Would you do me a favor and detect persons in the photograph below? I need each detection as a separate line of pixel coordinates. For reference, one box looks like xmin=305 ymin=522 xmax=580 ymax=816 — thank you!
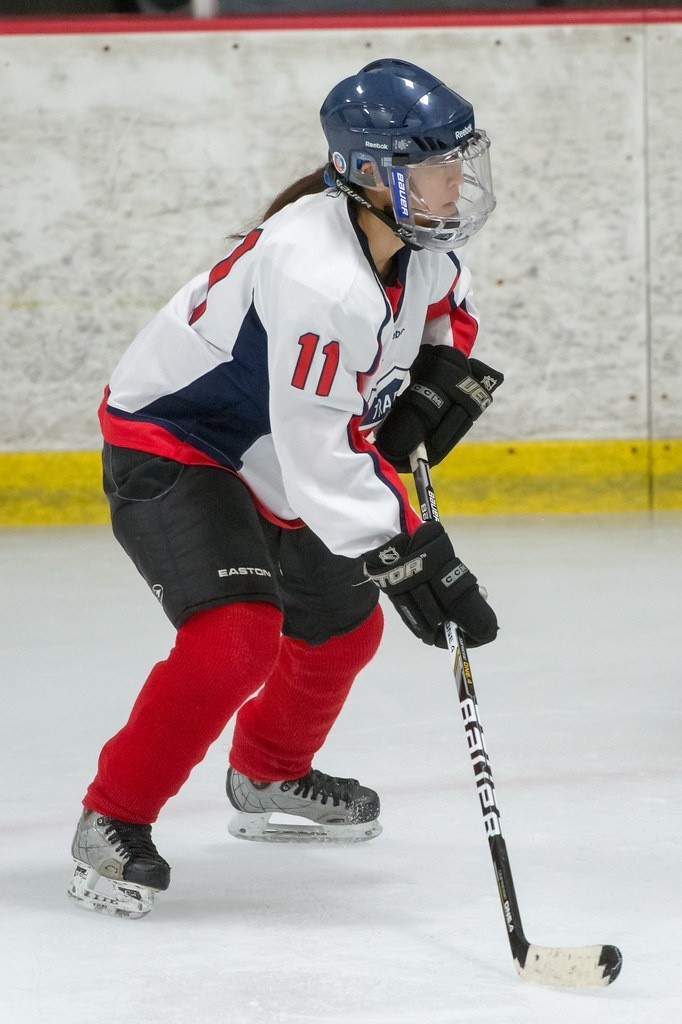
xmin=70 ymin=58 xmax=503 ymax=890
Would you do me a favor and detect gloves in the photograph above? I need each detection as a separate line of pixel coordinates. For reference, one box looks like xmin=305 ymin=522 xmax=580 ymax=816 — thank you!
xmin=373 ymin=344 xmax=505 ymax=475
xmin=364 ymin=522 xmax=499 ymax=649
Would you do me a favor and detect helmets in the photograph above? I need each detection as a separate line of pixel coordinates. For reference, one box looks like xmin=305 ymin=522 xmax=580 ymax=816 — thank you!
xmin=320 ymin=59 xmax=498 ymax=254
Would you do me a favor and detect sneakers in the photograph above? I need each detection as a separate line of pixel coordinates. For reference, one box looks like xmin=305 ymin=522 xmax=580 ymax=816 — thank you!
xmin=226 ymin=765 xmax=383 ymax=849
xmin=67 ymin=804 xmax=172 ymax=920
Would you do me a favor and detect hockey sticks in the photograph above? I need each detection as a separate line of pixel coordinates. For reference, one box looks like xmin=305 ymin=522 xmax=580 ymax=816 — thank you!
xmin=409 ymin=446 xmax=625 ymax=994
